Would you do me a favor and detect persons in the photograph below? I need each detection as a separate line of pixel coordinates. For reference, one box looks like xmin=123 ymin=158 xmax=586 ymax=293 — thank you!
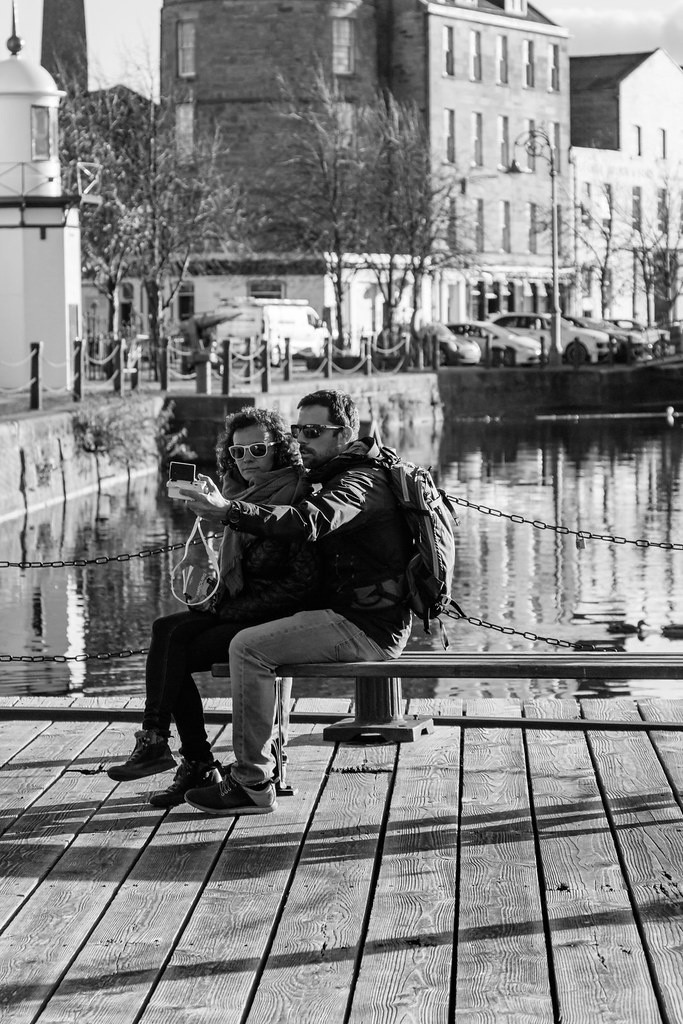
xmin=109 ymin=408 xmax=320 ymax=808
xmin=180 ymin=389 xmax=413 ymax=815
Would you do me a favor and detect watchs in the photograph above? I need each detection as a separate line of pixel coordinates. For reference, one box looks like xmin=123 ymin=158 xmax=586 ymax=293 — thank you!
xmin=226 ymin=501 xmax=240 ymax=526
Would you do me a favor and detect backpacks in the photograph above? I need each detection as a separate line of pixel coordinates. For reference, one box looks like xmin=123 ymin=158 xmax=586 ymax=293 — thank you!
xmin=378 ymin=444 xmax=468 ymax=653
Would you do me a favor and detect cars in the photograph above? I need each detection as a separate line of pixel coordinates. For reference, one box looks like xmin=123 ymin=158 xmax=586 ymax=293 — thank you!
xmin=564 ymin=316 xmax=653 ymax=361
xmin=447 ymin=322 xmax=543 ymax=366
xmin=491 ymin=313 xmax=618 ymax=364
xmin=609 ymin=319 xmax=672 ymax=359
xmin=364 ymin=323 xmax=481 ymax=366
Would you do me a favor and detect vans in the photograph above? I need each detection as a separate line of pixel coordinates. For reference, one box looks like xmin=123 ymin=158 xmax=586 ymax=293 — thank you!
xmin=219 ymin=298 xmax=333 ymax=373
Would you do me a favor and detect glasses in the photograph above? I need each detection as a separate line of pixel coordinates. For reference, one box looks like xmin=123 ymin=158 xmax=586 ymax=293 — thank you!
xmin=228 ymin=441 xmax=278 ymax=460
xmin=291 ymin=424 xmax=343 ymax=439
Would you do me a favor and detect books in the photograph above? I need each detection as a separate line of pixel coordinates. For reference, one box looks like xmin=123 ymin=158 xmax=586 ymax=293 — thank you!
xmin=182 ymin=565 xmax=224 ymax=614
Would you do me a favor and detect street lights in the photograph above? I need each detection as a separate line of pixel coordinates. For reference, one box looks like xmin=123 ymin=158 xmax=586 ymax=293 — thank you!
xmin=517 ymin=131 xmax=564 ymax=354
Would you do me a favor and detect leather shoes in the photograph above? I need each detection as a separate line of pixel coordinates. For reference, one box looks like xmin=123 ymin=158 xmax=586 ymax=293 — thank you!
xmin=108 ymin=732 xmax=177 ymax=781
xmin=150 ymin=758 xmax=223 ymax=808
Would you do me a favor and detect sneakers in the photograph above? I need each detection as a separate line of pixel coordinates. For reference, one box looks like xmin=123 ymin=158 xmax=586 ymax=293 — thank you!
xmin=184 ymin=762 xmax=273 ymax=816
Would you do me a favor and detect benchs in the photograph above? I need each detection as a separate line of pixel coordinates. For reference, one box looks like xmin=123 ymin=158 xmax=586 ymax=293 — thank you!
xmin=212 ymin=650 xmax=683 ymax=796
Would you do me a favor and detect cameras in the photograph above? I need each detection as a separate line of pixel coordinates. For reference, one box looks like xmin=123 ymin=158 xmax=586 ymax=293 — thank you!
xmin=165 ymin=461 xmax=208 ymax=502
